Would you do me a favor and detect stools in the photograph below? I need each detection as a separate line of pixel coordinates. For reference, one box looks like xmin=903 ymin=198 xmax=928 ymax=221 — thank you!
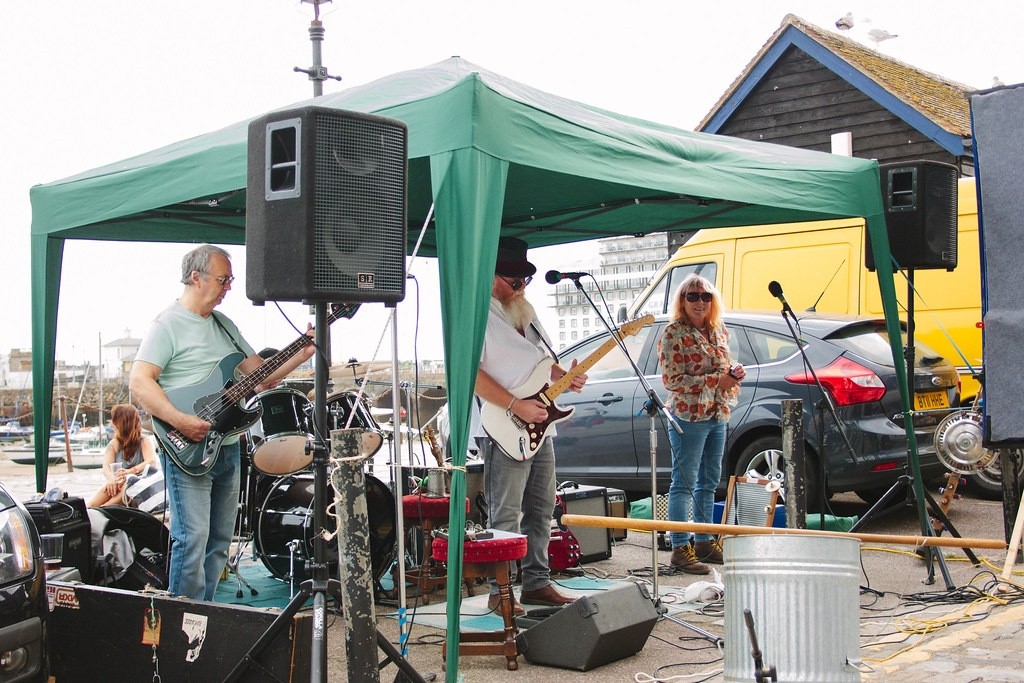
xmin=391 ymin=492 xmax=475 ymax=601
xmin=431 ymin=530 xmax=528 ymax=669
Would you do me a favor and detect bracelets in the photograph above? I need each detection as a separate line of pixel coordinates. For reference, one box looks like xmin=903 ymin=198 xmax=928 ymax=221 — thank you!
xmin=560 ymin=372 xmax=566 ymax=378
xmin=506 ymin=396 xmax=516 ymax=411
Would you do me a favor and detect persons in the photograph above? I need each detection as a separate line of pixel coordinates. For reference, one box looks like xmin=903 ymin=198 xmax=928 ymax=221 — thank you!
xmin=231 ymin=347 xmax=315 ymax=542
xmin=656 ymin=273 xmax=747 ymax=574
xmin=474 ymin=237 xmax=588 ymax=616
xmin=128 ymin=245 xmax=315 ymax=602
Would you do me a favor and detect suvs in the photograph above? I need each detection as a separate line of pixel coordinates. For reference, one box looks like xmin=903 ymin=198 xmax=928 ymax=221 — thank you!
xmin=435 ymin=310 xmax=965 ymax=514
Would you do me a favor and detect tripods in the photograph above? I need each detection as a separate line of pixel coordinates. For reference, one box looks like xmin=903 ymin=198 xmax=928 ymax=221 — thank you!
xmin=222 ymin=302 xmax=426 ymax=683
xmin=846 ymin=269 xmax=983 ymax=586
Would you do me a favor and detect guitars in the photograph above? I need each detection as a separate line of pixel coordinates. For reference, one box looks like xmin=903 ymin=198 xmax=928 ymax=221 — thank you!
xmin=150 ymin=303 xmax=363 ymax=478
xmin=480 ymin=310 xmax=656 ymax=463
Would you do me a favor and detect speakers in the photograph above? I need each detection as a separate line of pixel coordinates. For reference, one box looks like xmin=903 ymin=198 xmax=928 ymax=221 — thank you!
xmin=245 ymin=105 xmax=407 ymax=308
xmin=556 ymin=484 xmax=612 ymax=563
xmin=515 ymin=580 xmax=658 ymax=671
xmin=865 ymin=160 xmax=960 ymax=273
xmin=606 ymin=488 xmax=628 ymax=541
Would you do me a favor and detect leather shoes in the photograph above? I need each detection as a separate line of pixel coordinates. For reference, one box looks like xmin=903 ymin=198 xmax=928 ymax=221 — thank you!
xmin=520 ymin=584 xmax=578 ymax=607
xmin=487 ymin=590 xmax=525 ymax=616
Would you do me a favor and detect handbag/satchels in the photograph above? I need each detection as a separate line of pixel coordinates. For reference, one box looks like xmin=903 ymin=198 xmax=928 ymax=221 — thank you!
xmin=121 ymin=464 xmax=171 ymax=515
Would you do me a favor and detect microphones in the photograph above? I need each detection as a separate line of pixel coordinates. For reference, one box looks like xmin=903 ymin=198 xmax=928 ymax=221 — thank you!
xmin=545 ymin=270 xmax=589 ymax=284
xmin=768 ymin=281 xmax=798 ymax=322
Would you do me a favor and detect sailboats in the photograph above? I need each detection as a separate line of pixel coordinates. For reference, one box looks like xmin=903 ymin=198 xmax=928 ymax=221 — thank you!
xmin=1 ymin=332 xmax=159 ymax=470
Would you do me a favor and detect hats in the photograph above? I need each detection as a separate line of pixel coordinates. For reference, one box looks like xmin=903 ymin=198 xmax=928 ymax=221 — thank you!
xmin=495 ymin=236 xmax=537 ymax=278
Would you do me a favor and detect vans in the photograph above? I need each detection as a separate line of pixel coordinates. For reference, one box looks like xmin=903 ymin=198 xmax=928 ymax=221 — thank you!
xmin=618 ymin=176 xmax=1023 ymax=499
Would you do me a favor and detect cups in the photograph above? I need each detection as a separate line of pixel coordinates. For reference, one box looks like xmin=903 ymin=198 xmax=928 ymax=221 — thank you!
xmin=40 ymin=534 xmax=65 ymax=571
xmin=109 ymin=462 xmax=123 ymax=484
xmin=426 ymin=469 xmax=446 ymax=498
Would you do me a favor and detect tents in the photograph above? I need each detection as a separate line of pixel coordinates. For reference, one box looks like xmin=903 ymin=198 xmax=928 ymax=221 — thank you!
xmin=29 ymin=56 xmax=936 ymax=683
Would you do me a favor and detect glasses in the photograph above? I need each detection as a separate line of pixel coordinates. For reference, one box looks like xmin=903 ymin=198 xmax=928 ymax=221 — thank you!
xmin=199 ymin=270 xmax=235 ymax=286
xmin=496 ymin=272 xmax=533 ymax=290
xmin=683 ymin=291 xmax=712 ymax=303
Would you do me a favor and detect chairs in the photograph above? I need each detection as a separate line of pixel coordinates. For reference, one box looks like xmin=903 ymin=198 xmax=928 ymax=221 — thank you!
xmin=777 ymin=345 xmax=798 ymax=359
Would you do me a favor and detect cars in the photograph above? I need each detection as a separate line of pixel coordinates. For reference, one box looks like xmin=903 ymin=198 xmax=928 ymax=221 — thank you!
xmin=0 ymin=483 xmax=51 ymax=683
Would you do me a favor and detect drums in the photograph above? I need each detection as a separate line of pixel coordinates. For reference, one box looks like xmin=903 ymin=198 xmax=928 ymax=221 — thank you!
xmin=243 ymin=387 xmax=316 ymax=477
xmin=326 ymin=390 xmax=384 ymax=463
xmin=252 ymin=465 xmax=397 ymax=590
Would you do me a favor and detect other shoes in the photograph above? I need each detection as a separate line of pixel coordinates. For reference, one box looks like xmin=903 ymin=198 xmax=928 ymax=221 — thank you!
xmin=669 ymin=543 xmax=711 ymax=574
xmin=693 ymin=539 xmax=724 ymax=564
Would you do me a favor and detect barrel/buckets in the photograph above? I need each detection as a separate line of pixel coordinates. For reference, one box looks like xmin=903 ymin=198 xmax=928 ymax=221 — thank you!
xmin=722 ymin=533 xmax=875 ymax=683
